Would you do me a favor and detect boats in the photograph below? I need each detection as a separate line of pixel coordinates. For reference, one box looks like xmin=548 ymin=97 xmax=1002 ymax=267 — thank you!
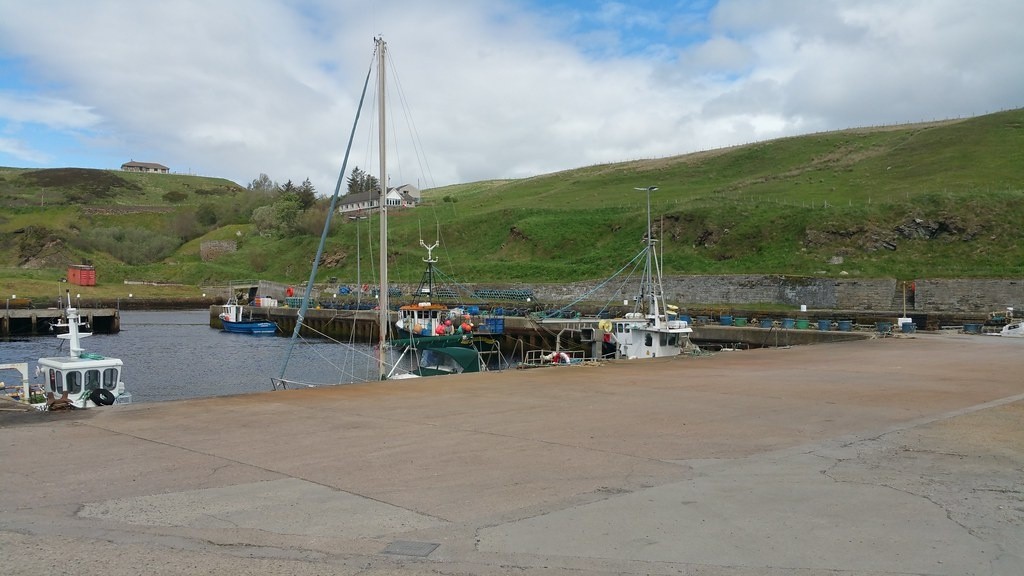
xmin=219 ymin=280 xmax=278 ymax=333
xmin=0 ymin=281 xmax=133 ymax=412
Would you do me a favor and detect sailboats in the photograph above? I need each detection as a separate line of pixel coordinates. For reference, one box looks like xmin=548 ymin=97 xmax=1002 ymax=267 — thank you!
xmin=269 ymin=34 xmax=749 ymax=390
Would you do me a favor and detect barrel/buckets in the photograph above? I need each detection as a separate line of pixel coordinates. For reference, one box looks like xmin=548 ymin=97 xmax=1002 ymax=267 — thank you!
xmin=818 ymin=320 xmax=832 ymax=331
xmin=679 ymin=316 xmax=690 ymax=324
xmin=838 ymin=320 xmax=852 ymax=331
xmin=796 ymin=320 xmax=809 ymax=329
xmin=720 ymin=317 xmax=732 ymax=326
xmin=782 ymin=319 xmax=794 ymax=328
xmin=964 ymin=323 xmax=980 ymax=332
xmin=759 ymin=319 xmax=772 ymax=328
xmin=735 ymin=318 xmax=747 ymax=327
xmin=902 ymin=322 xmax=916 ymax=332
xmin=878 ymin=321 xmax=891 ymax=332
